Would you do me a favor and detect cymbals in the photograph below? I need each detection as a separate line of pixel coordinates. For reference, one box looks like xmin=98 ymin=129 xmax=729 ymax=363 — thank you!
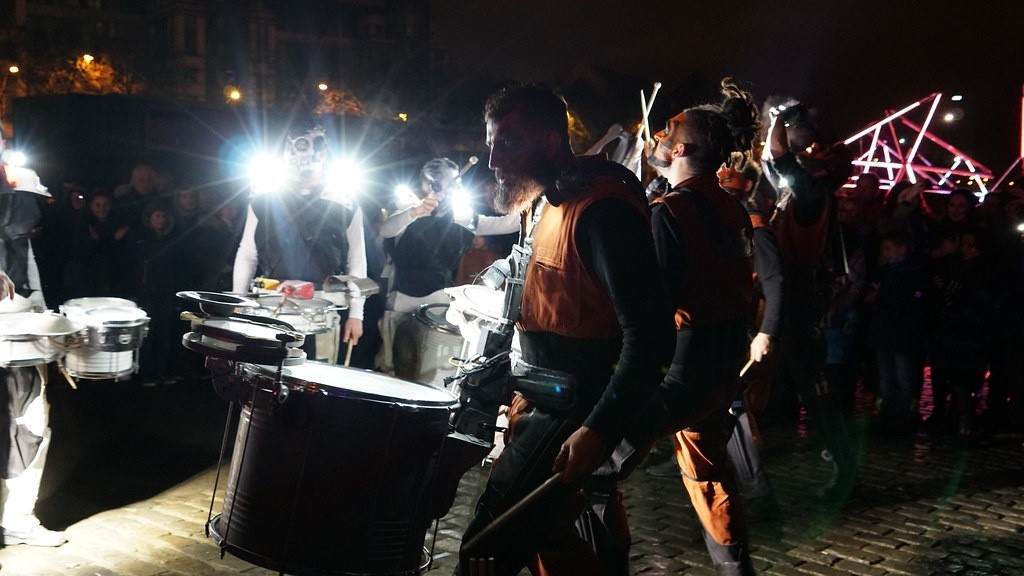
xmin=25 ymin=311 xmax=83 ymax=336
xmin=176 ymin=291 xmax=261 ymax=316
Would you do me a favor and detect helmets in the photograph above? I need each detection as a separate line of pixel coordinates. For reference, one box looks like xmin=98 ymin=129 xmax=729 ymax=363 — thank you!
xmin=1 ymin=166 xmax=51 ymax=197
xmin=422 ymin=158 xmax=463 ymax=194
xmin=285 ymin=124 xmax=332 ymax=180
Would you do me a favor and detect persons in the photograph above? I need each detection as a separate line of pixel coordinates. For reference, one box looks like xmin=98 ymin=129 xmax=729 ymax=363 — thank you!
xmin=452 ymin=84 xmax=678 ymax=576
xmin=0 ymin=77 xmax=1024 ymax=576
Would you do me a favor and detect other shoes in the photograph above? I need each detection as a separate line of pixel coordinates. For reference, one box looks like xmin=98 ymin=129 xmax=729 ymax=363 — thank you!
xmin=824 ymin=475 xmax=840 ymax=492
xmin=646 ymin=459 xmax=682 ymax=477
xmin=750 ymin=494 xmax=777 ymax=518
xmin=0 ymin=526 xmax=67 ymax=547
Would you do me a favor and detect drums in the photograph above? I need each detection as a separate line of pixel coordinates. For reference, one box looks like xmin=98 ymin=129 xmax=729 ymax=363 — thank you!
xmin=409 ymin=303 xmax=518 ymax=449
xmin=57 ymin=296 xmax=144 ymax=381
xmin=234 ymin=295 xmax=342 ymax=365
xmin=203 ymin=358 xmax=464 ymax=576
xmin=0 ymin=311 xmax=66 ymax=369
xmin=181 ymin=314 xmax=308 ymax=366
xmin=80 ymin=304 xmax=151 ymax=353
